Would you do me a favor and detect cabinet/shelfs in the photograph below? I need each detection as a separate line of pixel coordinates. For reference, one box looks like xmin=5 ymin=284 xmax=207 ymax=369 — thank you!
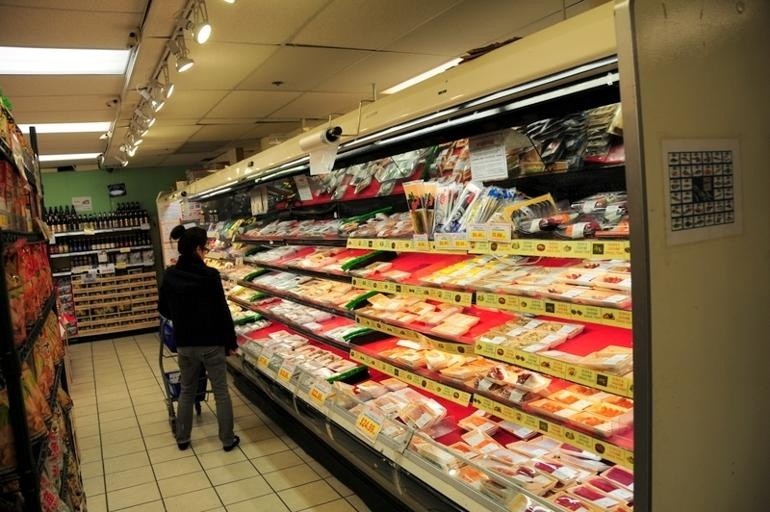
xmin=46 ymin=201 xmax=161 ymax=341
xmin=0 ymin=133 xmax=86 ymax=512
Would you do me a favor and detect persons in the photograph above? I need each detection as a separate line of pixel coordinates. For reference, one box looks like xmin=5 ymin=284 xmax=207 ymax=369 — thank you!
xmin=157 ymin=225 xmax=239 ymax=452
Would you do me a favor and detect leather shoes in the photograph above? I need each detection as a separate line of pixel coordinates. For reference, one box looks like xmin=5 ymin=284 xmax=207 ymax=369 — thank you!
xmin=222 ymin=434 xmax=240 ymax=452
xmin=177 ymin=440 xmax=192 ymax=450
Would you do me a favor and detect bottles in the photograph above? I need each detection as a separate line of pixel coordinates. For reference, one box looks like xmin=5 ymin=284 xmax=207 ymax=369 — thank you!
xmin=41 ymin=200 xmax=151 ymax=272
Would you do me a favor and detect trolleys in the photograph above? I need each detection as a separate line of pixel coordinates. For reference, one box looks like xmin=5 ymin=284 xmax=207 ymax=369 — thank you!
xmin=159 ymin=315 xmax=207 ymax=418
